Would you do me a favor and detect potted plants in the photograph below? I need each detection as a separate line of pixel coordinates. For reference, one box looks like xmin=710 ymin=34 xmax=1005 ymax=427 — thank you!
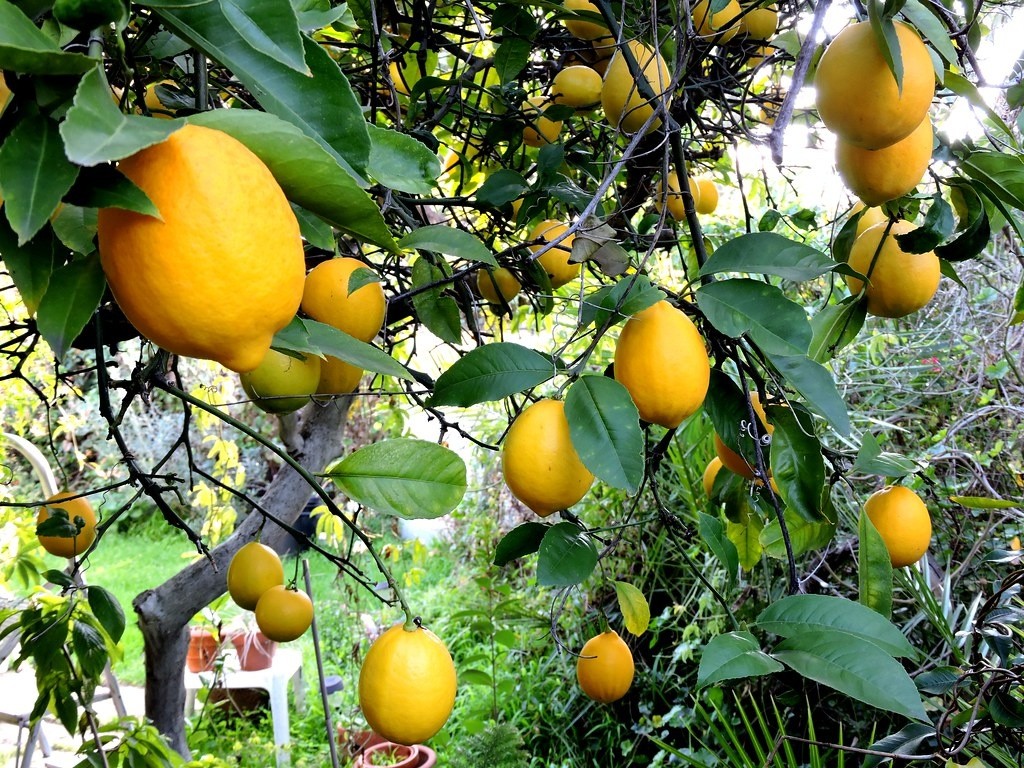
xmin=358 ymin=741 xmax=420 ymax=768
xmin=218 ymin=596 xmax=277 ymax=671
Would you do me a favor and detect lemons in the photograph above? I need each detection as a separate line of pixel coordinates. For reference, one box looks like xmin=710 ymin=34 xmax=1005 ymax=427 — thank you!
xmin=37 ymin=0 xmax=939 ymax=746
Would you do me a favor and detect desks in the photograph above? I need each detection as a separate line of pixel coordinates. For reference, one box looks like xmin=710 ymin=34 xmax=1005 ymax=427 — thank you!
xmin=184 ymin=648 xmax=305 ymax=768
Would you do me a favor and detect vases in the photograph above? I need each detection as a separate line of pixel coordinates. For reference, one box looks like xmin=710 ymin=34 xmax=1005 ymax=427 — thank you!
xmin=353 ymin=744 xmax=438 ymax=768
